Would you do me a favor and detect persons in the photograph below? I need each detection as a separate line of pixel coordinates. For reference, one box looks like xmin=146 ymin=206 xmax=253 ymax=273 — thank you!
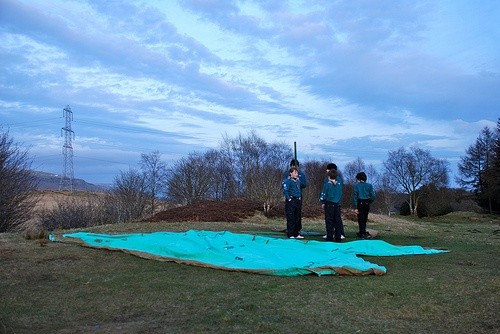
xmin=281 ymin=167 xmax=306 ymax=239
xmin=280 ymin=158 xmax=308 ymax=233
xmin=318 ymin=169 xmax=344 ymax=243
xmin=321 ymin=162 xmax=347 ymax=240
xmin=353 ymin=172 xmax=375 ymax=239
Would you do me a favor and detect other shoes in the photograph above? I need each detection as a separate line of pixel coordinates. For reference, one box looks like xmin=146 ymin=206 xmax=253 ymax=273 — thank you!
xmin=296 ymin=235 xmax=305 ymax=238
xmin=360 ymin=232 xmax=372 ymax=240
xmin=322 ymin=234 xmax=335 ymax=239
xmin=290 ymin=236 xmax=295 ymax=239
xmin=340 ymin=235 xmax=346 ymax=239
